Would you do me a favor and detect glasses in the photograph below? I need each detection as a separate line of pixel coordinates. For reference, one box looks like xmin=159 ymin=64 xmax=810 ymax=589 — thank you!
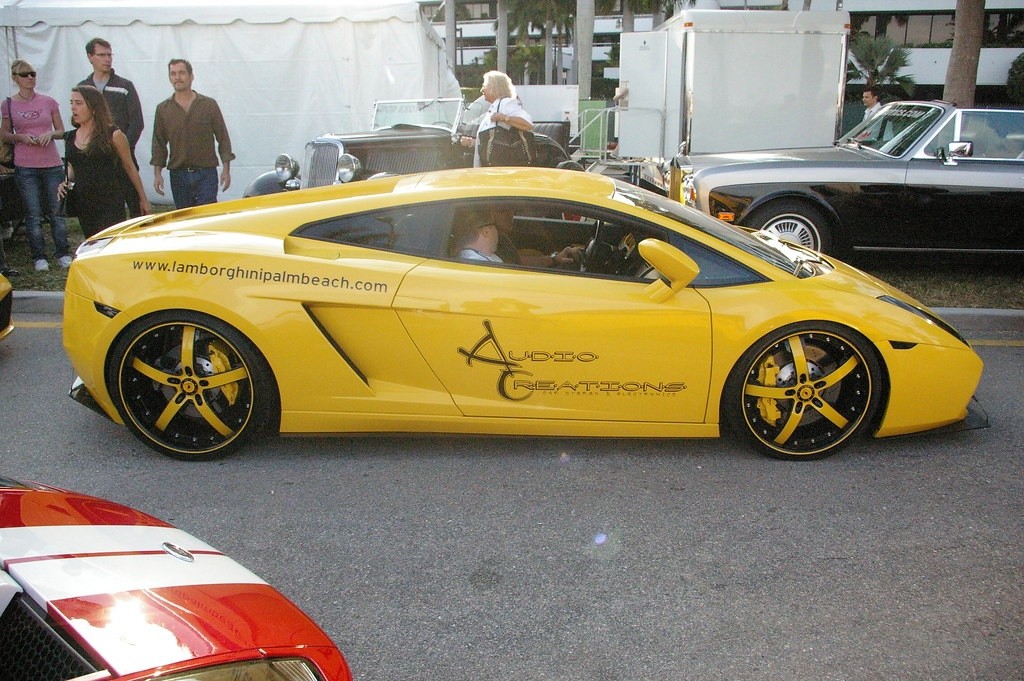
xmin=95 ymin=53 xmax=115 ymax=60
xmin=471 ymin=221 xmax=500 ymax=231
xmin=17 ymin=71 xmax=36 ymax=78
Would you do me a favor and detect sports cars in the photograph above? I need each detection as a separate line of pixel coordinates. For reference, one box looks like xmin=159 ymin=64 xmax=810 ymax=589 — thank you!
xmin=60 ymin=166 xmax=986 ymax=462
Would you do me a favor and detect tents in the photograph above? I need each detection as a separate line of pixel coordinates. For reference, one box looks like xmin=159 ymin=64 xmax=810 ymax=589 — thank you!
xmin=0 ymin=3 xmax=463 ymax=208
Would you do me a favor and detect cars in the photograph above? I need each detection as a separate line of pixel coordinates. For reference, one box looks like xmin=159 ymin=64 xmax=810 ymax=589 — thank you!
xmin=671 ymin=99 xmax=1023 ymax=264
xmin=299 ymin=120 xmax=571 ymax=190
xmin=0 ymin=470 xmax=351 ymax=680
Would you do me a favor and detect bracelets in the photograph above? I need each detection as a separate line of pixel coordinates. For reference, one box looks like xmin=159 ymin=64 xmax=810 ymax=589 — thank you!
xmin=468 ymin=137 xmax=474 ymax=148
xmin=11 ymin=134 xmax=17 ymax=144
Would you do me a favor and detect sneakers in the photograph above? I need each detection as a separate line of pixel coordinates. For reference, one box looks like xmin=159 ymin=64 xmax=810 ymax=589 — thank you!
xmin=58 ymin=255 xmax=73 ymax=269
xmin=33 ymin=259 xmax=50 ymax=272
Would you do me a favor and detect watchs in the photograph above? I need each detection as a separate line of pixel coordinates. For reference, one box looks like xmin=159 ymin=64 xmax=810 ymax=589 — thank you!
xmin=550 ymin=251 xmax=561 ymax=264
xmin=505 ymin=115 xmax=510 ymax=124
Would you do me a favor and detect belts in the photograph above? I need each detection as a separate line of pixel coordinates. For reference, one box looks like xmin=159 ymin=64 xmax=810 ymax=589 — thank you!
xmin=170 ymin=165 xmax=216 ymax=173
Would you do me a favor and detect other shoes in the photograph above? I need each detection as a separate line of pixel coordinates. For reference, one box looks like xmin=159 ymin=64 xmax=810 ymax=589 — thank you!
xmin=1 ymin=268 xmax=20 ymax=277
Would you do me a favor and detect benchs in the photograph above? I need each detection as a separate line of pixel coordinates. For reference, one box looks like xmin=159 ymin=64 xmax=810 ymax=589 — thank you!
xmin=457 ymin=120 xmax=571 ymax=152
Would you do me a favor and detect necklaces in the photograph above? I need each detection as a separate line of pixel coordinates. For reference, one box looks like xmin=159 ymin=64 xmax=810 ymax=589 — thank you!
xmin=18 ymin=91 xmax=37 ymax=102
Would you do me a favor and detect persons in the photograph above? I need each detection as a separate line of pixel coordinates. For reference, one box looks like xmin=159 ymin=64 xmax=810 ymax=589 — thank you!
xmin=863 ymin=87 xmax=893 ymax=143
xmin=461 ymin=70 xmax=532 ymax=168
xmin=452 ymin=201 xmax=582 ymax=266
xmin=0 ymin=38 xmax=236 ymax=271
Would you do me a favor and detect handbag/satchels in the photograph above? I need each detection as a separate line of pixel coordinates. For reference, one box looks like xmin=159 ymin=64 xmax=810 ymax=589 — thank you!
xmin=478 ymin=96 xmax=540 ymax=167
xmin=0 ymin=96 xmax=14 ymax=169
xmin=53 ymin=131 xmax=78 ymax=218
